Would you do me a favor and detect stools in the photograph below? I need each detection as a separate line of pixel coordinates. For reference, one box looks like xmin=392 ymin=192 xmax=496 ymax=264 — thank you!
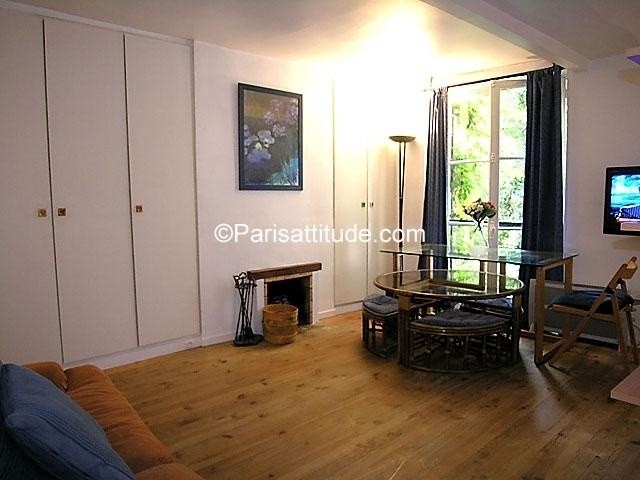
xmin=360 ymin=291 xmax=524 ymax=375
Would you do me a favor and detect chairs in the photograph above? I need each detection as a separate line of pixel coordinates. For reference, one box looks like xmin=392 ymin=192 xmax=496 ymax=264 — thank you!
xmin=542 ymin=256 xmax=640 ymax=375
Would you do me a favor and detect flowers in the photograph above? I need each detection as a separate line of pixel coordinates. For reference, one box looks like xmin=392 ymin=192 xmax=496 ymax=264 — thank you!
xmin=462 ymin=197 xmax=498 ymax=246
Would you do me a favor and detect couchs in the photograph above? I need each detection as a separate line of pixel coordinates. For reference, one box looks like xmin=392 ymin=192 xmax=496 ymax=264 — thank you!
xmin=0 ymin=361 xmax=205 ymax=480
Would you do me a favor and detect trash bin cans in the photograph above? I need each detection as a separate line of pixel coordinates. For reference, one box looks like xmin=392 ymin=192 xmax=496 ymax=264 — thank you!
xmin=262 ymin=304 xmax=298 ymax=344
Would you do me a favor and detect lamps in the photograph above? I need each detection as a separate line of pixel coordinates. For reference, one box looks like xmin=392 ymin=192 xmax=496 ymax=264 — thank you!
xmin=389 ymin=135 xmax=416 ymax=286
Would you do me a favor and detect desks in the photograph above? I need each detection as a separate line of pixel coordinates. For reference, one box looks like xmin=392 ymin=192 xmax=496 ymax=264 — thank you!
xmin=373 ymin=268 xmax=526 ymax=372
xmin=380 ymin=242 xmax=580 ymax=366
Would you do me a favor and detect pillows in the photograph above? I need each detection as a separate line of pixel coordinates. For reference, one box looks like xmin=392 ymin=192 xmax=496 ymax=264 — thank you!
xmin=2 ymin=362 xmax=135 ymax=480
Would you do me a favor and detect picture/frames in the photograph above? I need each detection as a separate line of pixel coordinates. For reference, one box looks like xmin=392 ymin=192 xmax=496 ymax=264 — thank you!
xmin=237 ymin=82 xmax=303 ymax=191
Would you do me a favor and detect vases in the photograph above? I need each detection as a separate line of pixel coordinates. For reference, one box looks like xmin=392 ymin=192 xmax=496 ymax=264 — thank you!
xmin=466 ymin=220 xmax=489 ymax=258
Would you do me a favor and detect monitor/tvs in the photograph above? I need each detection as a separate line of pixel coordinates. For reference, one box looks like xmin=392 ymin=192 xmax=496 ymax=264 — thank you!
xmin=602 ymin=165 xmax=640 ymax=237
